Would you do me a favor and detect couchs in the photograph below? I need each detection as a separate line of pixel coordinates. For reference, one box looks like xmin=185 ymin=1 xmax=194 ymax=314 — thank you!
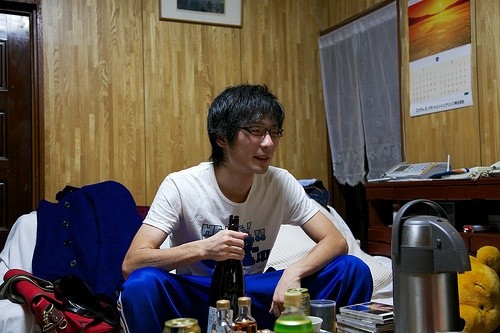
xmin=0 ymin=181 xmax=394 ymax=333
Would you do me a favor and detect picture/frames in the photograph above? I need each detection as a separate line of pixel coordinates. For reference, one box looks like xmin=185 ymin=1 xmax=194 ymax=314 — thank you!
xmin=159 ymin=0 xmax=243 ymax=28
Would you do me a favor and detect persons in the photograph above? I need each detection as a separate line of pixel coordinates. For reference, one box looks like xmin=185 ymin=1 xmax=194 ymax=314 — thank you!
xmin=116 ymin=83 xmax=374 ymax=332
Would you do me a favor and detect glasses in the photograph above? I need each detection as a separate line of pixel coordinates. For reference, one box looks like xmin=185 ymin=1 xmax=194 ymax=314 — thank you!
xmin=236 ymin=124 xmax=284 ymax=138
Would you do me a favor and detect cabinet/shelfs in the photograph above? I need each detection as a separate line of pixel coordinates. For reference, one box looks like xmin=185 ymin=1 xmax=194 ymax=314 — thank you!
xmin=365 ymin=177 xmax=500 ymax=258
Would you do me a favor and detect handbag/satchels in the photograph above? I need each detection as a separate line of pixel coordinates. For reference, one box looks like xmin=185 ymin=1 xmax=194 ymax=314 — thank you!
xmin=0 ymin=269 xmax=121 ymax=333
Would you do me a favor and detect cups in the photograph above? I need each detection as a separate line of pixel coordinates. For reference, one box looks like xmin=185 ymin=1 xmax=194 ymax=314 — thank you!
xmin=307 ymin=316 xmax=323 ymax=333
xmin=309 ymin=300 xmax=337 ymax=333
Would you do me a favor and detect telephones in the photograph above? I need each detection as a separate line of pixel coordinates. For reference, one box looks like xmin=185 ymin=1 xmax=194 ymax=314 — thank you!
xmin=470 ymin=160 xmax=500 ymax=180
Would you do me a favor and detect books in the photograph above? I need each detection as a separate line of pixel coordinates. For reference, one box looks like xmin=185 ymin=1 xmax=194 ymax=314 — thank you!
xmin=335 ymin=301 xmax=394 ymax=333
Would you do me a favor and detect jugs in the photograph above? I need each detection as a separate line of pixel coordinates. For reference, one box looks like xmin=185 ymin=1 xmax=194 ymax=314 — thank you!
xmin=389 ymin=198 xmax=473 ymax=333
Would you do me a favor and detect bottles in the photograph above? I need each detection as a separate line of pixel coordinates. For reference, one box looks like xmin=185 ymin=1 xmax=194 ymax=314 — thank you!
xmin=211 ymin=300 xmax=237 ymax=333
xmin=272 ymin=290 xmax=315 ymax=333
xmin=207 ymin=214 xmax=246 ymax=333
xmin=232 ymin=297 xmax=257 ymax=333
